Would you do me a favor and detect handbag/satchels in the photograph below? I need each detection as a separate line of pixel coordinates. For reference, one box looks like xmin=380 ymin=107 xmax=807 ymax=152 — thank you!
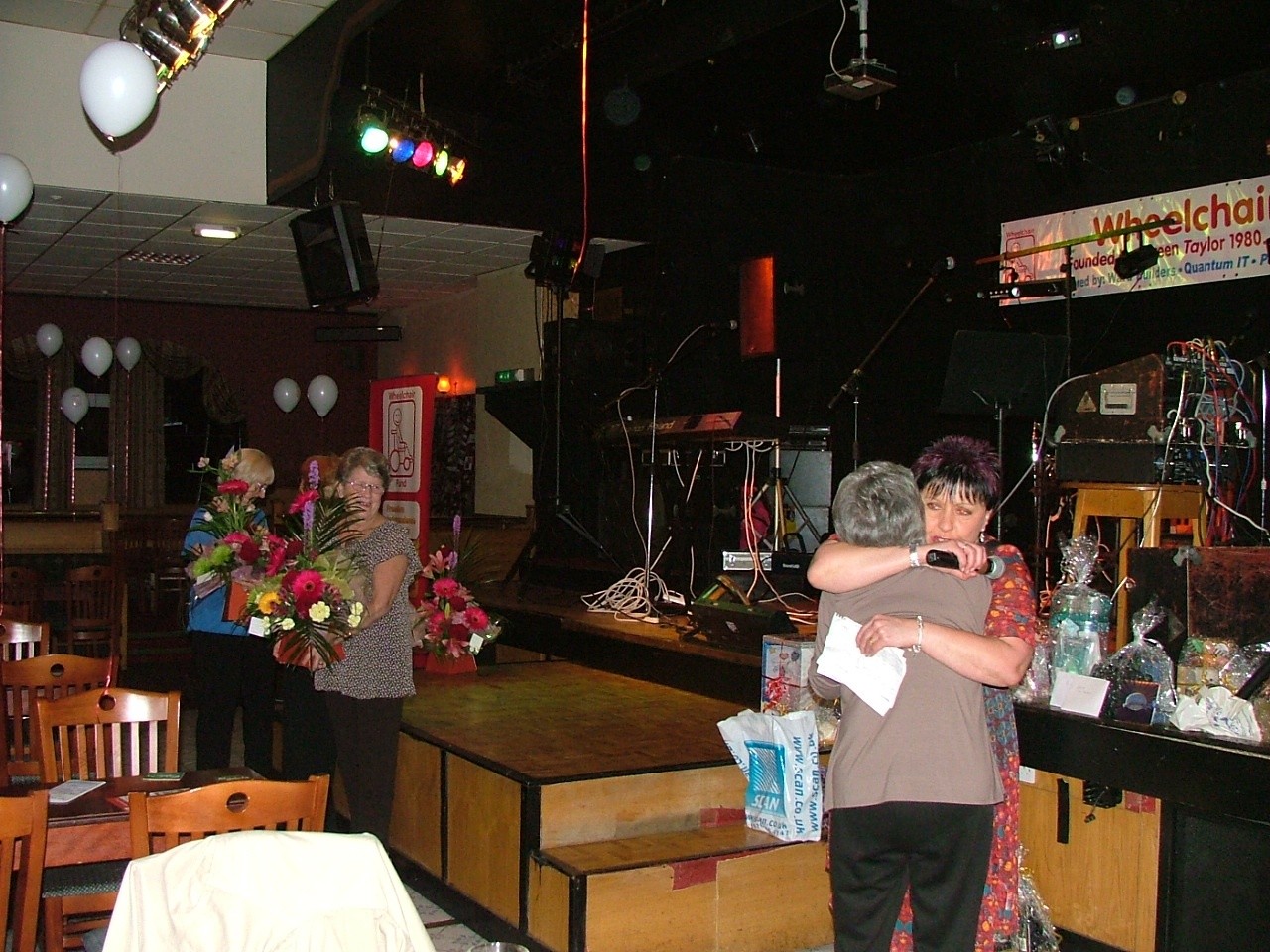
xmin=718 ymin=710 xmax=823 ymax=842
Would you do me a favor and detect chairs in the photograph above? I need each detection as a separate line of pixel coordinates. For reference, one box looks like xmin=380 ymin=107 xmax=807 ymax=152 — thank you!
xmin=0 ymin=526 xmax=330 ymax=952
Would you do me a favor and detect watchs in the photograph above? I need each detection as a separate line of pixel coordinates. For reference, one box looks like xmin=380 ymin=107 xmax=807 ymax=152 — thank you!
xmin=909 ymin=541 xmax=921 ymax=567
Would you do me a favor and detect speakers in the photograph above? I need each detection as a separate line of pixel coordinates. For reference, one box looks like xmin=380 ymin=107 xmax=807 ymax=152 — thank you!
xmin=291 ymin=200 xmax=381 ymax=310
xmin=765 ymin=445 xmax=833 ymax=555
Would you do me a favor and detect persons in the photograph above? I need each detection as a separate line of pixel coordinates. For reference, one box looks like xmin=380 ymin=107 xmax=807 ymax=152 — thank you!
xmin=280 ymin=448 xmax=425 ymax=850
xmin=182 ymin=449 xmax=276 ymax=771
xmin=806 ymin=437 xmax=1060 ymax=951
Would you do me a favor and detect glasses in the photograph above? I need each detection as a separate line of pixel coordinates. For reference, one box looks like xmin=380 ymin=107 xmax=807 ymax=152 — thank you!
xmin=344 ymin=480 xmax=386 ymax=496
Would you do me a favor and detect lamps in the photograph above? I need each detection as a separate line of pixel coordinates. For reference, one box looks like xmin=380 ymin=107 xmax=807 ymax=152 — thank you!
xmin=502 ymin=228 xmax=606 ymax=602
xmin=436 ymin=375 xmax=451 ymax=398
xmin=357 ymin=92 xmax=467 ymax=187
xmin=1114 ymin=232 xmax=1159 ymax=279
xmin=120 ymin=0 xmax=235 ymax=95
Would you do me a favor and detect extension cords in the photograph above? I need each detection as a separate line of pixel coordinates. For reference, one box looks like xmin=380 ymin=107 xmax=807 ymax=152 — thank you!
xmin=625 ymin=612 xmax=659 ymax=623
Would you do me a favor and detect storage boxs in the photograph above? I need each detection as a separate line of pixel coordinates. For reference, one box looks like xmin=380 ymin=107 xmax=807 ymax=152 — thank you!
xmin=761 ymin=633 xmax=841 ymax=745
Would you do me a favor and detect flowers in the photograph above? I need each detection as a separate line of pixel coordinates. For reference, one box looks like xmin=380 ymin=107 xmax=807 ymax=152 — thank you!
xmin=176 ymin=445 xmax=371 ymax=677
xmin=411 ymin=525 xmax=511 ymax=667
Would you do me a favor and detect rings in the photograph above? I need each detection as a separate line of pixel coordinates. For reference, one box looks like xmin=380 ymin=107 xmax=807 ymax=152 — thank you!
xmin=962 ymin=544 xmax=968 ymax=551
xmin=870 ymin=636 xmax=874 ymax=644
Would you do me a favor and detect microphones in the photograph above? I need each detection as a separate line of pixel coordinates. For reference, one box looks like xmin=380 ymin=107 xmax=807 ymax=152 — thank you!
xmin=927 ymin=550 xmax=1005 ymax=579
xmin=706 ymin=321 xmax=737 ymax=330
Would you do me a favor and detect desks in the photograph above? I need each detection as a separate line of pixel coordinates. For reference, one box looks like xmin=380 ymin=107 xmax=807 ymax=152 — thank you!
xmin=3 ymin=580 xmax=108 ymax=601
xmin=1057 ymin=480 xmax=1209 ymax=651
xmin=6 ymin=685 xmax=85 ymax=720
xmin=0 ymin=766 xmax=268 ymax=871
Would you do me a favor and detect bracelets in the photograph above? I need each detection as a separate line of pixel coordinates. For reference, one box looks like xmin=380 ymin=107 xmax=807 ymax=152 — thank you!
xmin=907 ymin=615 xmax=923 ymax=655
